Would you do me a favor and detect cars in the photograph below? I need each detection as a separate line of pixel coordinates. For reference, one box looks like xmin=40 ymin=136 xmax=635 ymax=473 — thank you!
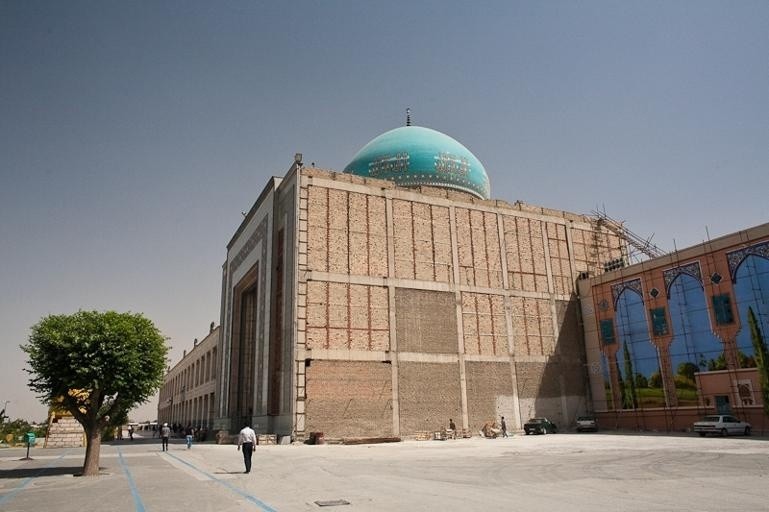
xmin=693 ymin=414 xmax=752 ymax=436
xmin=523 ymin=417 xmax=557 ymax=435
xmin=575 ymin=414 xmax=599 ymax=432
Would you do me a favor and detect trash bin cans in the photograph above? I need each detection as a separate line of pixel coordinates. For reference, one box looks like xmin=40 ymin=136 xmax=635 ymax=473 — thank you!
xmin=24 ymin=432 xmax=35 ymax=447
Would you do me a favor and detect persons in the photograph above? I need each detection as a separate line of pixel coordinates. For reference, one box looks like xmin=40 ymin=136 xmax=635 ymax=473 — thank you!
xmin=236 ymin=420 xmax=257 ymax=476
xmin=449 ymin=418 xmax=457 ymax=439
xmin=500 ymin=416 xmax=508 ymax=438
xmin=127 ymin=421 xmax=202 ymax=452
